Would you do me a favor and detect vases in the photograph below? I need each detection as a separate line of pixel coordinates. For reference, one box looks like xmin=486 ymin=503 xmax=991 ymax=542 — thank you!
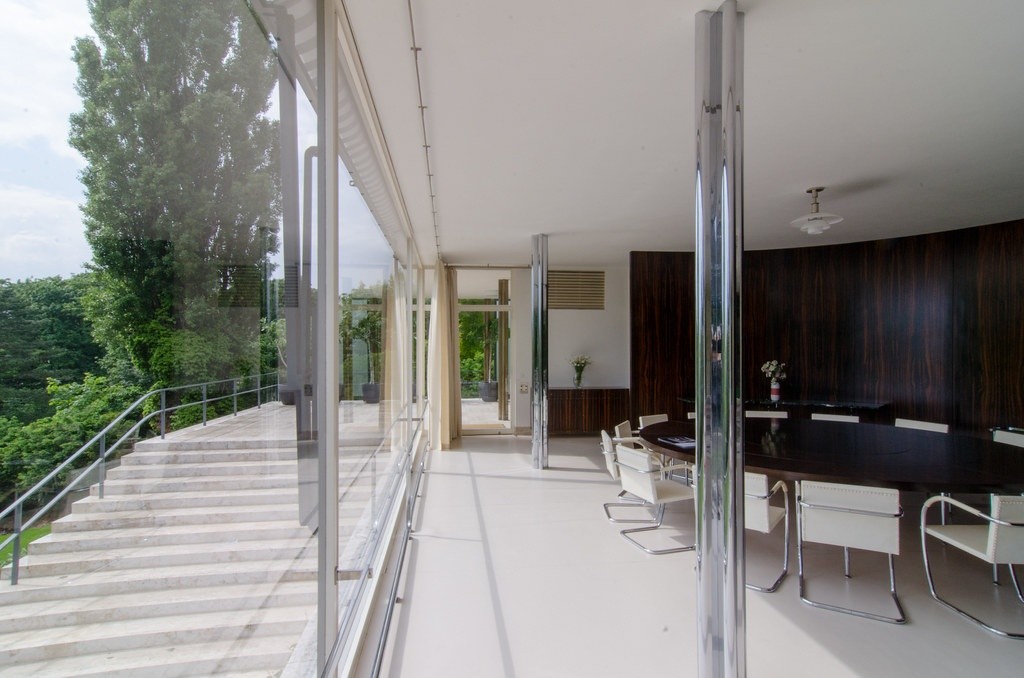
xmin=571 ymin=366 xmax=585 ymax=387
xmin=770 ymin=382 xmax=781 ymax=401
xmin=278 ymin=385 xmax=300 ymax=406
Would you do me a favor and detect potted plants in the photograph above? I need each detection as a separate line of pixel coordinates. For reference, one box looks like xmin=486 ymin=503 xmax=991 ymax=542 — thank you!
xmin=350 ymin=313 xmax=382 ymax=403
xmin=470 ymin=320 xmax=498 ymax=402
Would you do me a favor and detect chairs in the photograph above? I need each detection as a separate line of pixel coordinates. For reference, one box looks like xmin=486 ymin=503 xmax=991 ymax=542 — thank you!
xmin=602 ymin=411 xmax=1024 ymax=640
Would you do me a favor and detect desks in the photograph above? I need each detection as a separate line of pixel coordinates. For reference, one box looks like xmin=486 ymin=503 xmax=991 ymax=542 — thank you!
xmin=639 ymin=418 xmax=1024 ymax=496
xmin=531 ymin=387 xmax=631 ymax=437
xmin=676 ymin=392 xmax=893 ymax=425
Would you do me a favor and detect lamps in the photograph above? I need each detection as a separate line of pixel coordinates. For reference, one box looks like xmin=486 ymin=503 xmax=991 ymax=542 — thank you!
xmin=790 ymin=187 xmax=845 ymax=235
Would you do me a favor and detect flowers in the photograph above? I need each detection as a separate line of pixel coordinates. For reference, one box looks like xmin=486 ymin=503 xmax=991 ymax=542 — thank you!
xmin=567 ymin=353 xmax=593 ymax=384
xmin=760 ymin=361 xmax=787 ymax=381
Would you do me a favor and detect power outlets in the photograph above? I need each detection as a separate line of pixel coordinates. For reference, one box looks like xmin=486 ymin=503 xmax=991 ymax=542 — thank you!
xmin=520 ymin=384 xmax=529 ymax=394
